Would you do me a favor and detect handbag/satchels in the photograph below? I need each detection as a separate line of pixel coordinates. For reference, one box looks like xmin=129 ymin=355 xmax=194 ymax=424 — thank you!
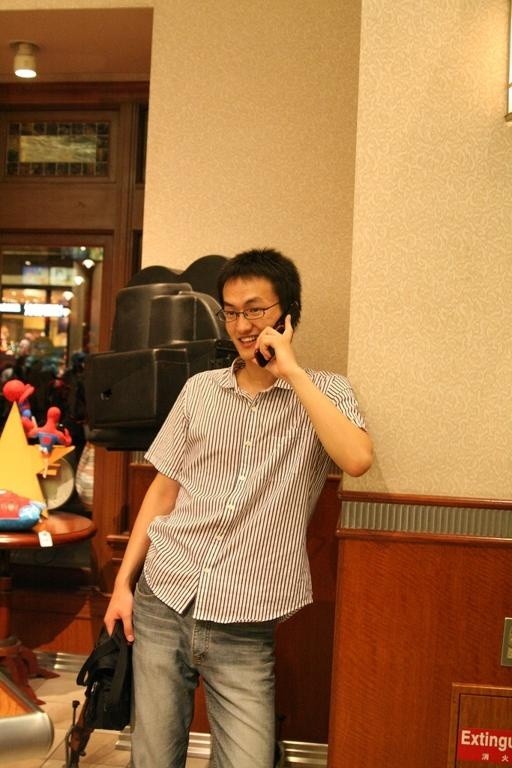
xmin=76 ymin=619 xmax=134 ymax=731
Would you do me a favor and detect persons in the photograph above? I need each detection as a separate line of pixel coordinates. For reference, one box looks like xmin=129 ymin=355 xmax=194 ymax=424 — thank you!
xmin=0 ymin=339 xmax=87 ymax=439
xmin=103 ymin=250 xmax=372 ymax=768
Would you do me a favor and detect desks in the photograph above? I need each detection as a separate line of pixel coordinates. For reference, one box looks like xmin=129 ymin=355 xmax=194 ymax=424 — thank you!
xmin=0 ymin=509 xmax=98 ymax=705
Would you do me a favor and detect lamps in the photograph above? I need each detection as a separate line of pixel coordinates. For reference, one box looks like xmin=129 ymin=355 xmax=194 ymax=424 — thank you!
xmin=13 ymin=43 xmax=38 ymax=78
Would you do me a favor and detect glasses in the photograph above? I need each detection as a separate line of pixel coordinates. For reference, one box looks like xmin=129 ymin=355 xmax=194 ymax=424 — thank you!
xmin=215 ymin=300 xmax=281 ymax=323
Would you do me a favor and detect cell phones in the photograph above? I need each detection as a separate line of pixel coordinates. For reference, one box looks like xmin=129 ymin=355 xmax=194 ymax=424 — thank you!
xmin=256 ymin=303 xmax=300 ymax=367
